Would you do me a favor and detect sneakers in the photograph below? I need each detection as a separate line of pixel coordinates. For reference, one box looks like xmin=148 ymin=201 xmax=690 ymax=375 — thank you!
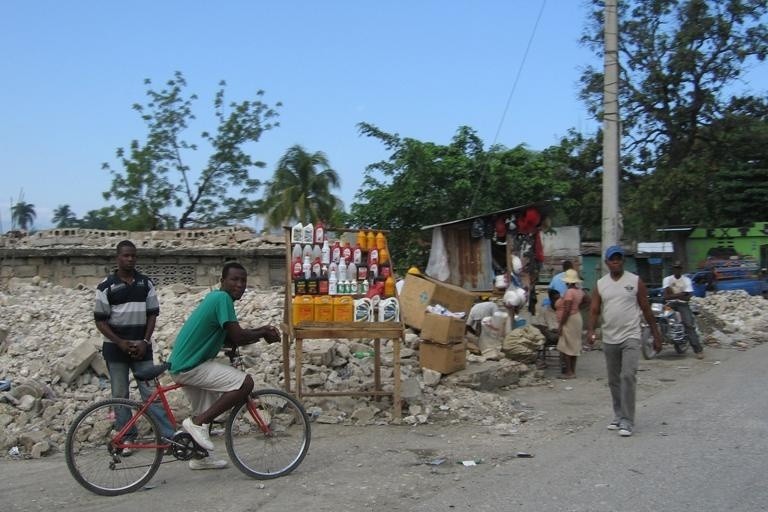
xmin=619 ymin=422 xmax=632 ymax=436
xmin=189 ymin=457 xmax=228 ymax=470
xmin=183 ymin=416 xmax=215 ymax=451
xmin=122 ymin=441 xmax=132 ymax=456
xmin=608 ymin=422 xmax=620 ymax=430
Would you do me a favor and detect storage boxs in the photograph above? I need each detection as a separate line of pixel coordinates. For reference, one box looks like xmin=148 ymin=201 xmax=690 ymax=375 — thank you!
xmin=417 ymin=341 xmax=467 ymax=374
xmin=398 ymin=269 xmax=477 ymax=329
xmin=420 ymin=311 xmax=467 ymax=343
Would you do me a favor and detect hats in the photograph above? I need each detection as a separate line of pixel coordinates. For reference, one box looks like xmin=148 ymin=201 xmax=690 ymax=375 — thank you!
xmin=605 ymin=246 xmax=625 ymax=258
xmin=561 ymin=269 xmax=583 ymax=284
xmin=672 ymin=260 xmax=683 ymax=269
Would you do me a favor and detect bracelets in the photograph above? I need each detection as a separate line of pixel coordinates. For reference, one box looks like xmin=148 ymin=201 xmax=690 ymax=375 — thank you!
xmin=144 ymin=338 xmax=149 ymax=343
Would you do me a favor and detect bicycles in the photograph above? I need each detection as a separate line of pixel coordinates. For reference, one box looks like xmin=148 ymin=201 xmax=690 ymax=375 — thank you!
xmin=64 ymin=325 xmax=310 ymax=495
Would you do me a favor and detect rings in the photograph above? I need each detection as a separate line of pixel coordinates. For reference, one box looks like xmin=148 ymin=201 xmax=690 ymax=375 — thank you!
xmin=591 ymin=335 xmax=596 ymax=341
xmin=128 ymin=352 xmax=131 ymax=355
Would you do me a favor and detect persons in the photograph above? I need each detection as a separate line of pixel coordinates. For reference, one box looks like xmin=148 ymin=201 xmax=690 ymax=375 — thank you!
xmin=93 ymin=240 xmax=177 ymax=457
xmin=661 ymin=260 xmax=704 ymax=359
xmin=585 ymin=246 xmax=662 ymax=437
xmin=550 ymin=260 xmax=573 ymax=295
xmin=538 ymin=288 xmax=562 ymax=346
xmin=553 ymin=268 xmax=586 ymax=379
xmin=167 ymin=261 xmax=283 ymax=470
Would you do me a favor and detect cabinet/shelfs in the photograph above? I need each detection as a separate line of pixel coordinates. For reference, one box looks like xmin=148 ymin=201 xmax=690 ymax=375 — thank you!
xmin=279 ymin=224 xmax=406 ymax=420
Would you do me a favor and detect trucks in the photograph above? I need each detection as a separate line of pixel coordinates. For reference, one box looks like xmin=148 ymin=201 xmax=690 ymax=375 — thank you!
xmin=647 ymin=255 xmax=767 ymax=302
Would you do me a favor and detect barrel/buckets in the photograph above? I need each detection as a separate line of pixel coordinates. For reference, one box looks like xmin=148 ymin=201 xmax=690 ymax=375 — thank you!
xmin=293 ymin=293 xmax=401 ymax=328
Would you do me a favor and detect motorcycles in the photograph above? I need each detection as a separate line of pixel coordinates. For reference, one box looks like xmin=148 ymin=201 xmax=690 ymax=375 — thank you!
xmin=639 ymin=297 xmax=697 ymax=360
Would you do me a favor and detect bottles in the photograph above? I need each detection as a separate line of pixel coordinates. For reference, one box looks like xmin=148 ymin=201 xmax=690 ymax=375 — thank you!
xmin=352 ymin=351 xmax=375 ymax=359
xmin=288 ymin=219 xmax=395 ymax=298
